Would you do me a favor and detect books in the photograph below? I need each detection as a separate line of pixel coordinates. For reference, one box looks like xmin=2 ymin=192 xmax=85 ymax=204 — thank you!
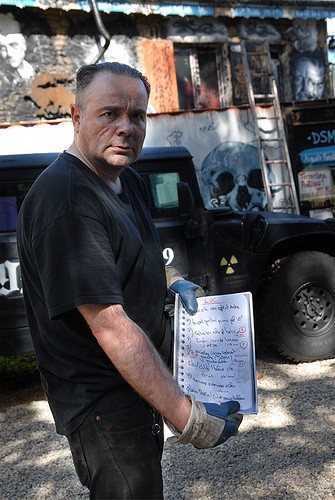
xmin=172 ymin=292 xmax=258 ymax=414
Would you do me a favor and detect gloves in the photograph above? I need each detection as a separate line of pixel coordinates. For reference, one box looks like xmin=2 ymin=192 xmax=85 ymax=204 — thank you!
xmin=165 ymin=265 xmax=206 ymax=317
xmin=162 ymin=393 xmax=243 ymax=450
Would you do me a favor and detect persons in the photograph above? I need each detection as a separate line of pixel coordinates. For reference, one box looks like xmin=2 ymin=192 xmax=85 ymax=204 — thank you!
xmin=14 ymin=62 xmax=243 ymax=500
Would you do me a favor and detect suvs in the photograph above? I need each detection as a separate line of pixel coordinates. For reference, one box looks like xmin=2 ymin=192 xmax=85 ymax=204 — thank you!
xmin=0 ymin=144 xmax=335 ymax=364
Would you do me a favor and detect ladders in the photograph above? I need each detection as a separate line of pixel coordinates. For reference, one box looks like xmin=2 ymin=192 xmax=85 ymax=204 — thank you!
xmin=240 ymin=38 xmax=301 ymax=217
